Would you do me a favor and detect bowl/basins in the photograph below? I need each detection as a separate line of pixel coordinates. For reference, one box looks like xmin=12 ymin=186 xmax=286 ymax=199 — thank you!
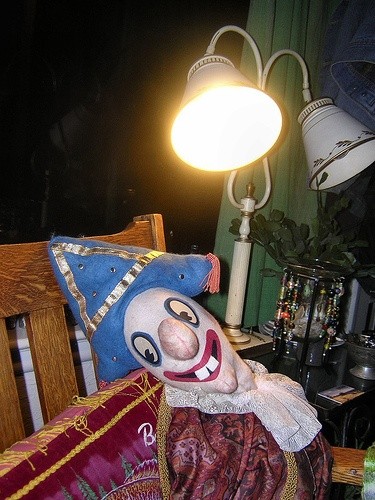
xmin=343 ymin=334 xmax=375 ymax=380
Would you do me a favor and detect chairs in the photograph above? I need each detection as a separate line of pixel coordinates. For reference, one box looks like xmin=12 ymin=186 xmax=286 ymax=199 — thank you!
xmin=0 ymin=213 xmax=375 ymax=500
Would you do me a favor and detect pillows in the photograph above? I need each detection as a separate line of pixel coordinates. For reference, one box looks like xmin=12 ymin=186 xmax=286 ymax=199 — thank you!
xmin=0 ymin=367 xmax=166 ymax=500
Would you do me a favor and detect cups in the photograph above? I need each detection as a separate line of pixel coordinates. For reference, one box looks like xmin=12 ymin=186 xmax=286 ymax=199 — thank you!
xmin=272 ymin=263 xmax=315 ymax=365
xmin=301 ymin=258 xmax=347 ymax=367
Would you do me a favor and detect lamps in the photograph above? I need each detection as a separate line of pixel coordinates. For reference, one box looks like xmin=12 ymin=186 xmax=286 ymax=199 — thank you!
xmin=170 ymin=25 xmax=375 ymax=345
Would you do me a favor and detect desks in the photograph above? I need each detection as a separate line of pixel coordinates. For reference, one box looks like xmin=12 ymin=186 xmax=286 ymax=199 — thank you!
xmin=242 ymin=325 xmax=375 ymax=500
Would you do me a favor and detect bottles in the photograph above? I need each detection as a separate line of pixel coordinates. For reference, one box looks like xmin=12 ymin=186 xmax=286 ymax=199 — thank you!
xmin=361 ymin=442 xmax=375 ymax=500
